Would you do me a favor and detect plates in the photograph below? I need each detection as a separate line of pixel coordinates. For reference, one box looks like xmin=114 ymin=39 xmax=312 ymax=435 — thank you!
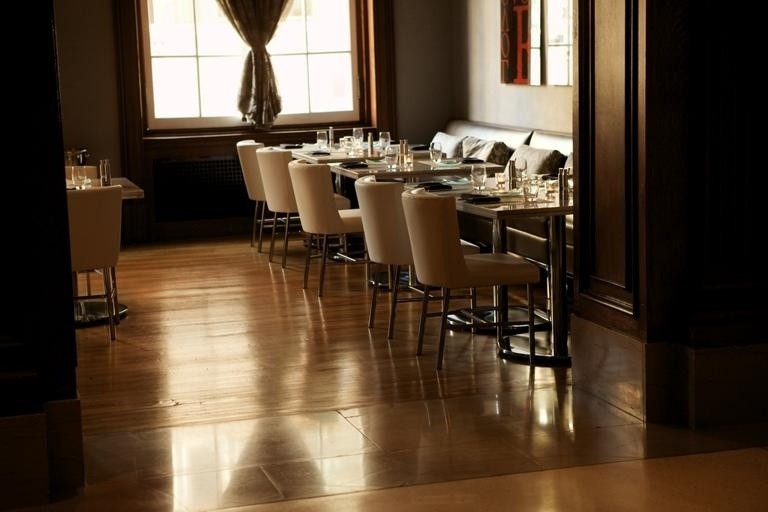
xmin=391 ymin=137 xmax=486 ymax=165
xmin=280 ymin=142 xmax=370 ymax=170
xmin=417 ymin=181 xmax=503 ymax=203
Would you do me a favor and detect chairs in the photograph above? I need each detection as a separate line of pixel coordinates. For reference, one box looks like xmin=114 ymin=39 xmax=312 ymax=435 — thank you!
xmin=402 ymin=186 xmax=541 ymax=381
xmin=67 ymin=159 xmax=148 ymax=338
xmin=230 ymin=116 xmax=574 ymax=299
xmin=355 ymin=176 xmax=476 ymax=343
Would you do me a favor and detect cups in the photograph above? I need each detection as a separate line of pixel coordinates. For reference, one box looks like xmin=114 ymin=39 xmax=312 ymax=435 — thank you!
xmin=468 ymin=156 xmax=574 ymax=205
xmin=316 ymin=126 xmax=464 ymax=169
xmin=72 ymin=167 xmax=86 ymax=187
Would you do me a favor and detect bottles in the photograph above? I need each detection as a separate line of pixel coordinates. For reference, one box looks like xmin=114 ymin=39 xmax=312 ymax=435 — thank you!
xmin=99 ymin=159 xmax=111 ymax=186
